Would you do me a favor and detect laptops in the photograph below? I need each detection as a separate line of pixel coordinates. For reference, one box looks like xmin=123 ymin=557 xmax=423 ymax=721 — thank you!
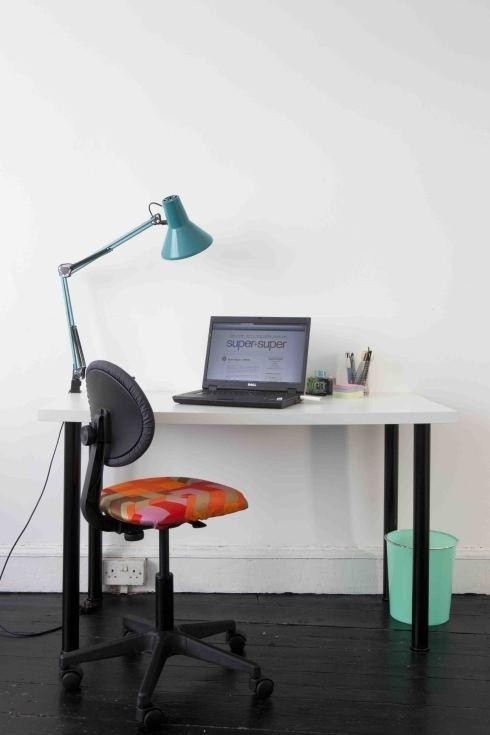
xmin=173 ymin=316 xmax=311 ymax=408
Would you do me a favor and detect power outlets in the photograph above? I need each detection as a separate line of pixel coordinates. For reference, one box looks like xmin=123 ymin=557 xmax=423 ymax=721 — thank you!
xmin=102 ymin=557 xmax=147 ymax=587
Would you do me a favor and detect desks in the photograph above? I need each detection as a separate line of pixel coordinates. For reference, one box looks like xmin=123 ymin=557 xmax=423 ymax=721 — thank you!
xmin=35 ymin=393 xmax=456 ymax=660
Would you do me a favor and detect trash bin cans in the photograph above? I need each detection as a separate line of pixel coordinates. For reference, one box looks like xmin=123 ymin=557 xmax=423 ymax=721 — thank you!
xmin=384 ymin=529 xmax=459 ymax=626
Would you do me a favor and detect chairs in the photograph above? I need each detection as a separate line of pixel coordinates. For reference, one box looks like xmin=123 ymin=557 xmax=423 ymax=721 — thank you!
xmin=60 ymin=360 xmax=274 ymax=732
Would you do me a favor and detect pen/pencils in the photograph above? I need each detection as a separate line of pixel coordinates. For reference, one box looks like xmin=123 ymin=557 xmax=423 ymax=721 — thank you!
xmin=346 ymin=351 xmax=375 ymax=384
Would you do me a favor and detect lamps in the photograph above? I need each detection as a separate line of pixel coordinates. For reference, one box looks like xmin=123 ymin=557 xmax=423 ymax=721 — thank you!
xmin=56 ymin=196 xmax=212 ymax=389
